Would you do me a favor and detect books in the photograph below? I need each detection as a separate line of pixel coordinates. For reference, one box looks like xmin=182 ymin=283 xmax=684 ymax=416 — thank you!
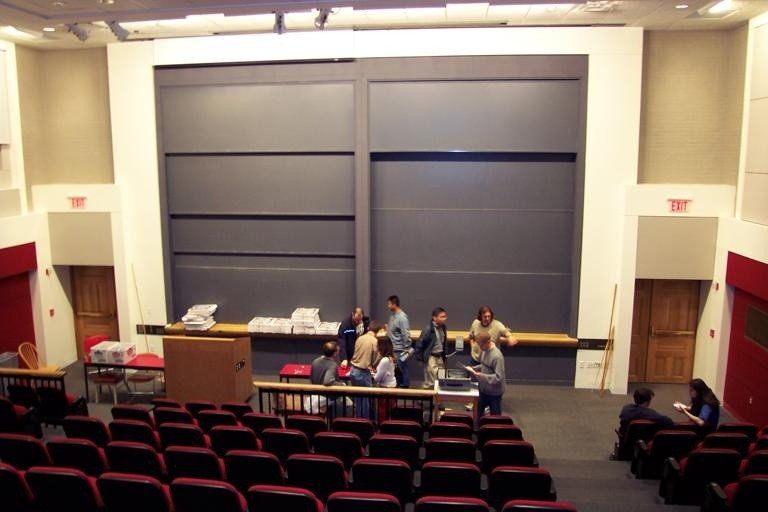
xmin=178 ymin=302 xmax=216 ymax=333
xmin=245 ymin=305 xmax=342 ymax=335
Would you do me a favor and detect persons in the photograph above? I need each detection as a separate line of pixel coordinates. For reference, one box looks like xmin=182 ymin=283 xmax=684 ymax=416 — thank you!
xmin=382 ymin=293 xmax=414 ymax=388
xmin=367 ymin=335 xmax=401 ymax=421
xmin=673 ymin=376 xmax=720 ymax=439
xmin=467 ymin=303 xmax=519 ymax=414
xmin=349 ymin=319 xmax=381 ymax=421
xmin=309 ymin=339 xmax=353 ymax=416
xmin=337 ymin=303 xmax=371 ymax=363
xmin=415 ymin=305 xmax=450 ymax=420
xmin=617 ymin=387 xmax=675 ymax=443
xmin=466 ymin=329 xmax=506 ymax=428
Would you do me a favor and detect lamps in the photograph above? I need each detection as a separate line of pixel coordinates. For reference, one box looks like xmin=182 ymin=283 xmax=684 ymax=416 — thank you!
xmin=313 ymin=7 xmax=332 ymax=31
xmin=104 ymin=21 xmax=132 ymax=42
xmin=66 ymin=22 xmax=91 ymax=42
xmin=271 ymin=9 xmax=289 ymax=37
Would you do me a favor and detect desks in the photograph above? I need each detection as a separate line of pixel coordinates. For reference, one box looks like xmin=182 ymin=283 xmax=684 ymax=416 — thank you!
xmin=163 ymin=319 xmax=581 ymax=346
